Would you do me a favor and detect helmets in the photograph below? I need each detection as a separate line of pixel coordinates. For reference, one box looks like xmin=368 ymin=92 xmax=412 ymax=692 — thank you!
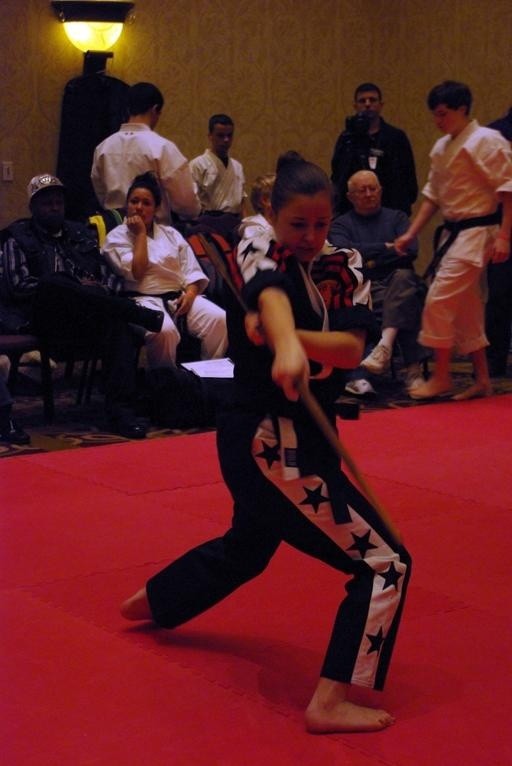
xmin=26 ymin=173 xmax=63 ymax=206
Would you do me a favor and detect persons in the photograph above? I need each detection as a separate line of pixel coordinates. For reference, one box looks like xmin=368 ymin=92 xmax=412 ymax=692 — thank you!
xmin=329 ymin=170 xmax=428 ymax=396
xmin=330 ymin=82 xmax=419 ymax=217
xmin=121 ymin=149 xmax=412 ymax=734
xmin=91 ymin=82 xmax=201 ymax=225
xmin=0 ymin=361 xmax=32 ymax=448
xmin=481 ymin=106 xmax=512 ymax=379
xmin=249 ymin=174 xmax=376 ymax=401
xmin=100 ymin=168 xmax=228 ymax=370
xmin=0 ymin=172 xmax=162 ymax=439
xmin=394 ymin=79 xmax=511 ymax=401
xmin=186 ymin=114 xmax=248 ymax=244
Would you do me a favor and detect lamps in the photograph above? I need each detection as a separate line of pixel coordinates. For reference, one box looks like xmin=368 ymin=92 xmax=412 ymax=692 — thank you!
xmin=51 ymin=0 xmax=134 ymax=54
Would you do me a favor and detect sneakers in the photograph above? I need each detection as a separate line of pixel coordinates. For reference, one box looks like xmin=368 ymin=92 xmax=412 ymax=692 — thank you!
xmin=345 ymin=379 xmax=377 ymax=395
xmin=360 ymin=345 xmax=393 ymax=374
xmin=397 ymin=363 xmax=425 ymax=391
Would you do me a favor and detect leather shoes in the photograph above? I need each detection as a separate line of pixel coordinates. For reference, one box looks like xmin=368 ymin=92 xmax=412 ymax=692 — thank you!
xmin=1 ymin=419 xmax=31 ymax=446
xmin=134 ymin=307 xmax=163 ymax=332
xmin=123 ymin=422 xmax=144 ymax=437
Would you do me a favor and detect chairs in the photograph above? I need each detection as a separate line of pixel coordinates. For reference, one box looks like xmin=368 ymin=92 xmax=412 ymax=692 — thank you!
xmin=0 ymin=333 xmax=94 ymax=423
